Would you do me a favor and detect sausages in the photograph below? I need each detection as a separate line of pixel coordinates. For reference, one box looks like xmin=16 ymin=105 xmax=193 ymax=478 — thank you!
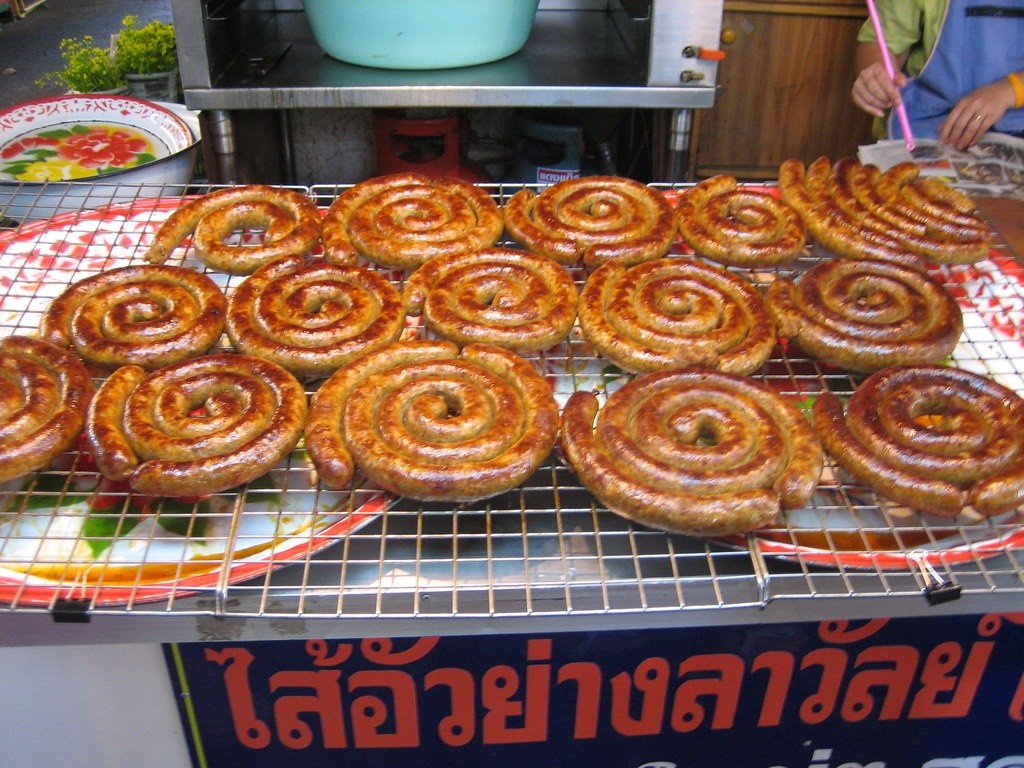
xmin=0 ymin=157 xmax=1024 ymax=531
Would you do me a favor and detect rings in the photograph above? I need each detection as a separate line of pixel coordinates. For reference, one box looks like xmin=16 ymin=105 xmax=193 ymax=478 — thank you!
xmin=973 ymin=113 xmax=983 ymax=122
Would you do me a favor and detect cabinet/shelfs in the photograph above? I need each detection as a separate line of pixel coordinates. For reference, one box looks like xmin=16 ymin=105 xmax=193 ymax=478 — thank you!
xmin=692 ymin=1 xmax=875 ymax=178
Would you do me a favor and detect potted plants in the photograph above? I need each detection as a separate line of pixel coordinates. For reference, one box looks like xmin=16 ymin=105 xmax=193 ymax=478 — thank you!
xmin=113 ymin=13 xmax=180 ymax=103
xmin=35 ymin=33 xmax=130 ymax=98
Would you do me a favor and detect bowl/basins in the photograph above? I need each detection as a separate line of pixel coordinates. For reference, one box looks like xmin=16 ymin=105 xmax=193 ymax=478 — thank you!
xmin=0 ymin=97 xmax=202 ymax=220
xmin=305 ymin=0 xmax=536 ymax=69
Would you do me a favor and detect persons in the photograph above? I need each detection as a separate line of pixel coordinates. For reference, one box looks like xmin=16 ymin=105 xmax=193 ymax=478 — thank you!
xmin=847 ymin=0 xmax=1024 ymax=162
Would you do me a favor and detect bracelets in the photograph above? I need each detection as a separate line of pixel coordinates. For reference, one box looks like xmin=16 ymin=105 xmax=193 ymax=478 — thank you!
xmin=1008 ymin=73 xmax=1023 ymax=110
